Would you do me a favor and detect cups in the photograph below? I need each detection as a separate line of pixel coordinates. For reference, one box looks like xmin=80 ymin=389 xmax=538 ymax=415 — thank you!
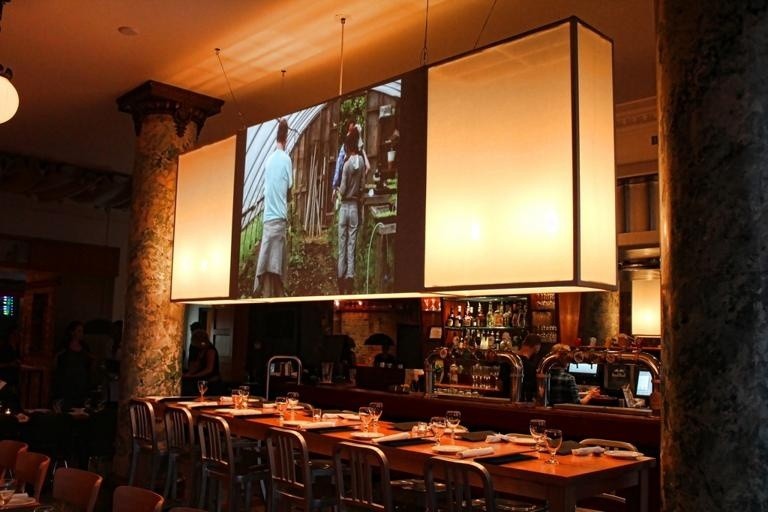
xmin=313 ymin=409 xmax=321 ymax=421
xmin=530 ymin=419 xmax=545 ymax=449
xmin=232 ymin=390 xmax=242 ymax=409
xmin=417 ymin=422 xmax=427 ymax=436
xmin=446 ymin=411 xmax=461 ymax=440
xmin=239 ymin=386 xmax=249 ymax=402
xmin=430 ymin=417 xmax=445 ymax=445
xmin=198 ymin=380 xmax=208 ymax=399
xmin=275 ymin=397 xmax=287 ymax=421
xmin=359 ymin=407 xmax=373 ymax=432
xmin=543 ymin=429 xmax=562 ymax=464
xmin=369 ymin=402 xmax=383 ymax=428
xmin=287 ymin=392 xmax=299 ymax=415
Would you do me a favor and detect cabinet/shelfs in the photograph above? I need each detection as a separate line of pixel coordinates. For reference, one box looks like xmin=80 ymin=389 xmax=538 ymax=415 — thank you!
xmin=420 ymin=297 xmax=582 ymax=353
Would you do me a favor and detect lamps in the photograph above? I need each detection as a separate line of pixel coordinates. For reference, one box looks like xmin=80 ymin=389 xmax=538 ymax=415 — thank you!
xmin=0 ymin=75 xmax=22 ymax=130
xmin=629 ymin=270 xmax=661 ymax=335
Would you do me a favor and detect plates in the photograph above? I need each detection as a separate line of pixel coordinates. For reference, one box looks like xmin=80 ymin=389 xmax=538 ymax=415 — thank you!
xmin=215 ymin=409 xmax=231 ymax=413
xmin=604 ymin=451 xmax=644 ymax=459
xmin=345 ymin=416 xmax=361 ymax=420
xmin=0 ymin=495 xmax=35 ymax=507
xmin=282 ymin=420 xmax=310 ymax=426
xmin=248 ymin=399 xmax=259 ymax=402
xmin=432 ymin=445 xmax=467 ymax=454
xmin=287 ymin=407 xmax=304 ymax=409
xmin=350 ymin=432 xmax=384 ymax=439
xmin=511 ymin=438 xmax=537 ymax=445
xmin=177 ymin=402 xmax=192 ymax=405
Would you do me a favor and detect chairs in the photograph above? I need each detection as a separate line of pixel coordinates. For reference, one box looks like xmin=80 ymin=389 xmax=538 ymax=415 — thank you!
xmin=162 ymin=405 xmax=195 ymax=507
xmin=0 ymin=439 xmax=29 ymax=469
xmin=53 ymin=466 xmax=103 ymax=512
xmin=423 ymin=453 xmax=495 ymax=512
xmin=330 ymin=439 xmax=393 ymax=512
xmin=16 ymin=452 xmax=50 ymax=502
xmin=126 ymin=396 xmax=169 ymax=490
xmin=263 ymin=425 xmax=348 ymax=511
xmin=197 ymin=413 xmax=270 ymax=511
xmin=112 ymin=485 xmax=165 ymax=512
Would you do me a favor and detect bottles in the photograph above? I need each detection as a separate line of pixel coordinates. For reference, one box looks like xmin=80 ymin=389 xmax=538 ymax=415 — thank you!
xmin=469 ymin=306 xmax=475 ymax=321
xmin=463 ymin=301 xmax=471 ymax=327
xmin=486 ymin=301 xmax=494 ymax=327
xmin=475 ymin=303 xmax=486 ymax=327
xmin=495 ymin=297 xmax=529 ymax=327
xmin=454 ymin=305 xmax=462 ymax=327
xmin=450 ymin=329 xmax=527 ymax=350
xmin=449 ymin=353 xmax=460 ymax=385
xmin=447 ymin=306 xmax=454 ymax=327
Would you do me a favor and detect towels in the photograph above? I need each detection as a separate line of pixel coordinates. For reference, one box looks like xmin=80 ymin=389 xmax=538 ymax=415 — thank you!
xmin=296 ymin=422 xmax=335 ymax=430
xmin=219 ymin=396 xmax=233 ymax=402
xmin=457 ymin=447 xmax=494 ymax=458
xmin=231 ymin=410 xmax=262 ymax=416
xmin=371 ymin=432 xmax=409 ymax=444
xmin=322 ymin=413 xmax=360 ymax=420
xmin=571 ymin=445 xmax=606 ymax=456
xmin=484 ymin=433 xmax=507 ymax=443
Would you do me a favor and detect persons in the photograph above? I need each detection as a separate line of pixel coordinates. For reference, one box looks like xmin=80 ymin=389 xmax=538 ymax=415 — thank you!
xmin=426 ymin=353 xmax=438 ymax=372
xmin=545 ymin=343 xmax=574 ymax=405
xmin=106 ymin=320 xmax=121 ymax=402
xmin=254 ymin=119 xmax=293 ymax=299
xmin=332 ymin=123 xmax=370 ymax=201
xmin=0 ymin=328 xmax=22 ymax=404
xmin=183 ymin=330 xmax=219 ymax=382
xmin=59 ymin=321 xmax=90 ymax=362
xmin=502 ymin=333 xmax=542 ymax=401
xmin=334 ymin=117 xmax=371 ymax=296
xmin=188 ymin=322 xmax=205 ymax=366
xmin=373 ymin=345 xmax=396 ymax=365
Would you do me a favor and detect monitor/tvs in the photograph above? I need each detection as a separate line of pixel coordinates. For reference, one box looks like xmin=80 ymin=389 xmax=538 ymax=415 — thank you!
xmin=634 ymin=367 xmax=653 ymax=397
xmin=568 ymin=363 xmax=598 ymax=376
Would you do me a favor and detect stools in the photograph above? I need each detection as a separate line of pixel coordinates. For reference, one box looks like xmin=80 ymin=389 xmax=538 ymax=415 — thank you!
xmin=14 ymin=364 xmax=44 ymax=408
